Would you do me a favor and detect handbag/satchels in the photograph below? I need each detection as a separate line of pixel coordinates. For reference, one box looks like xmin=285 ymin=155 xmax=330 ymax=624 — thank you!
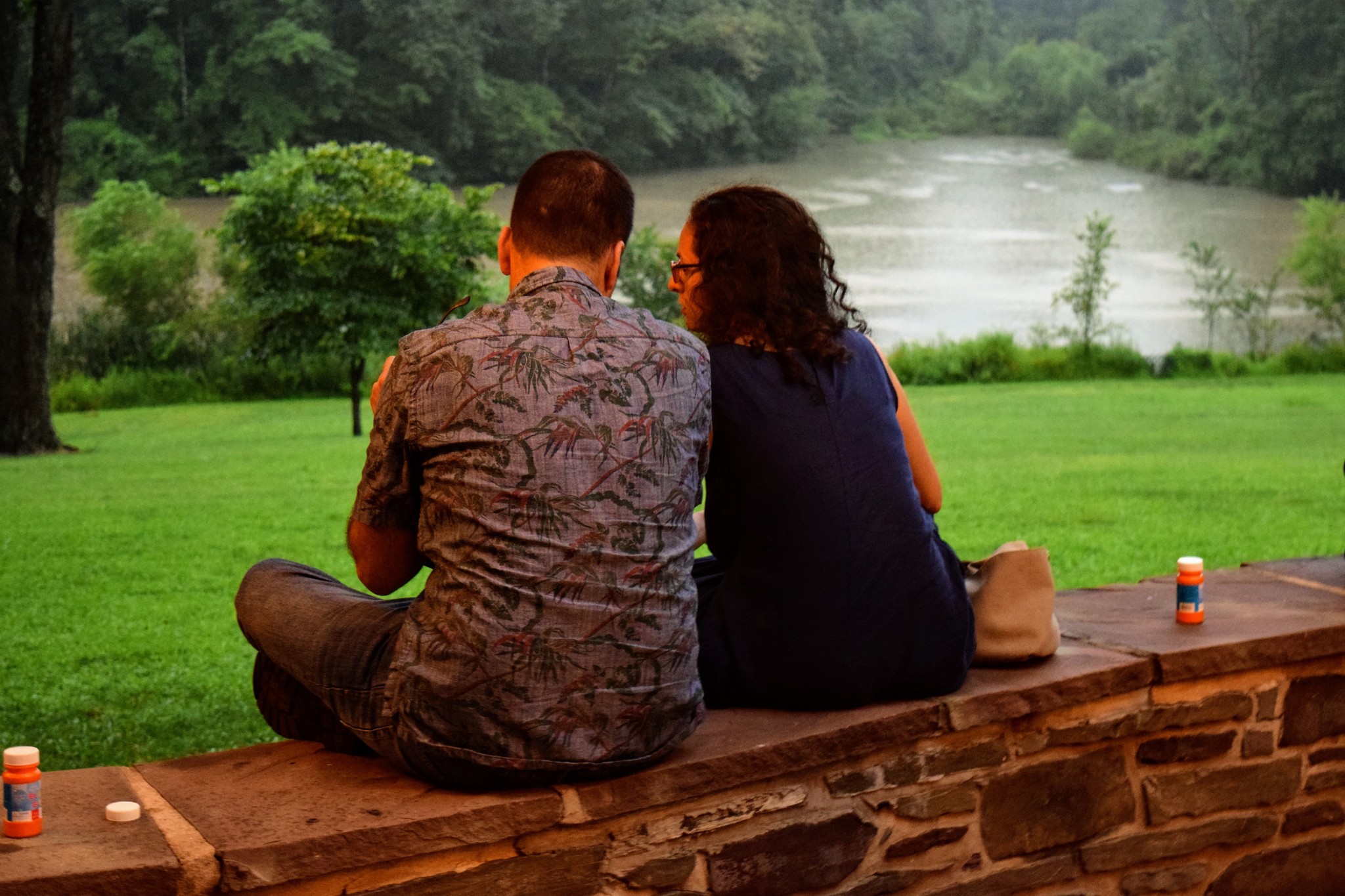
xmin=960 ymin=539 xmax=1060 ymax=660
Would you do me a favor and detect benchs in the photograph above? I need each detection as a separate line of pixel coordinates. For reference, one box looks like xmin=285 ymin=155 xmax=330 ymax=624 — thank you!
xmin=0 ymin=557 xmax=1345 ymax=896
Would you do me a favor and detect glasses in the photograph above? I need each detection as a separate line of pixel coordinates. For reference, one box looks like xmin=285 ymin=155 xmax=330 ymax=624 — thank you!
xmin=671 ymin=260 xmax=700 ymax=285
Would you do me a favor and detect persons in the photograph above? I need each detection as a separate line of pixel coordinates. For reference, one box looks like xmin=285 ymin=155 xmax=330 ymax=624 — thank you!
xmin=232 ymin=147 xmax=711 ymax=790
xmin=661 ymin=183 xmax=981 ymax=715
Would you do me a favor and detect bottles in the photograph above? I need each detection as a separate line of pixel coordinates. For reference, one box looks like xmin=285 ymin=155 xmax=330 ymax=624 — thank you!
xmin=1176 ymin=556 xmax=1204 ymax=624
xmin=3 ymin=746 xmax=43 ymax=837
